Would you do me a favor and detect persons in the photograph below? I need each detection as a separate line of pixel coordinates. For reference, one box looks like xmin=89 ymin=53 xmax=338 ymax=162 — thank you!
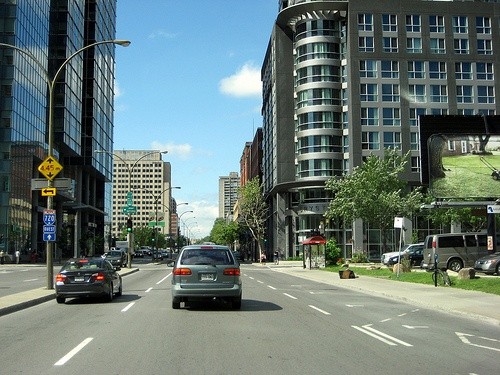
xmin=261 ymin=250 xmax=267 ymax=264
xmin=15 ymin=249 xmax=20 ymax=264
xmin=273 ymin=249 xmax=279 ymax=265
xmin=0 ymin=250 xmax=5 ymax=264
xmin=30 ymin=249 xmax=37 ymax=264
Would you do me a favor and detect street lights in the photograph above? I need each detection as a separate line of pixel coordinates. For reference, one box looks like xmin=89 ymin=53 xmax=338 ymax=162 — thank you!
xmin=162 ymin=202 xmax=200 ymax=252
xmin=135 ymin=183 xmax=182 ymax=261
xmin=94 ymin=150 xmax=168 ymax=266
xmin=0 ymin=39 xmax=132 ymax=290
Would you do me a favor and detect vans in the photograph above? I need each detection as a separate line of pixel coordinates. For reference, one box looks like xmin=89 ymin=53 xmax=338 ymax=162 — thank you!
xmin=422 ymin=233 xmax=490 ymax=272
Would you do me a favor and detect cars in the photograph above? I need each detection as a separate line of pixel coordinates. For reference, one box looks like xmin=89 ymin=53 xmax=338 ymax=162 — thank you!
xmin=381 ymin=243 xmax=425 ymax=267
xmin=474 ymin=249 xmax=500 ymax=275
xmin=134 ymin=246 xmax=169 ymax=260
xmin=104 ymin=250 xmax=128 ymax=269
xmin=55 ymin=257 xmax=123 ymax=304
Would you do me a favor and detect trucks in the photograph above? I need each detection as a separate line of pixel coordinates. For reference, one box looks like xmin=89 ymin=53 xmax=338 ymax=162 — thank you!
xmin=115 ymin=240 xmax=129 ymax=255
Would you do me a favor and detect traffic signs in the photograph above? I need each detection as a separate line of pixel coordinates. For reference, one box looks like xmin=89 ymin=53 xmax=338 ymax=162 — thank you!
xmin=122 ymin=205 xmax=137 ymax=215
xmin=127 ymin=192 xmax=133 ymax=207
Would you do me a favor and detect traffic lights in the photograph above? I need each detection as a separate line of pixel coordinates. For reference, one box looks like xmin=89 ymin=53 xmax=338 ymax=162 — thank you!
xmin=127 ymin=218 xmax=132 ymax=233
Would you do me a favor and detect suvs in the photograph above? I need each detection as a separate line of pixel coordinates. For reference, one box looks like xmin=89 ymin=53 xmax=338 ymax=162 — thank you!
xmin=171 ymin=245 xmax=242 ymax=309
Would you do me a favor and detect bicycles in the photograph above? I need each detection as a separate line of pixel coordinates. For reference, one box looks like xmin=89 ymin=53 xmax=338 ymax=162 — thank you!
xmin=431 ymin=253 xmax=453 ymax=286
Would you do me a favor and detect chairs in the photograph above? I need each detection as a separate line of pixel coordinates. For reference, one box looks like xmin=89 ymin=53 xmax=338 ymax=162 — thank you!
xmin=71 ymin=266 xmax=77 ymax=269
xmin=90 ymin=265 xmax=97 ymax=268
